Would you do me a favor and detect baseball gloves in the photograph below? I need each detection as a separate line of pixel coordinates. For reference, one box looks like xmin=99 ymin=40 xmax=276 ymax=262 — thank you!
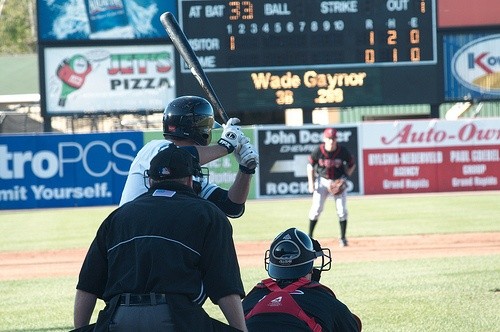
xmin=331 ymin=178 xmax=347 ymax=195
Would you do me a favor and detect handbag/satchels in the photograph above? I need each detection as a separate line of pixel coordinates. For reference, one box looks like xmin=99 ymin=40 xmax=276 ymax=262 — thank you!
xmin=167 ymin=293 xmax=214 ymax=332
xmin=70 ymin=295 xmax=120 ymax=332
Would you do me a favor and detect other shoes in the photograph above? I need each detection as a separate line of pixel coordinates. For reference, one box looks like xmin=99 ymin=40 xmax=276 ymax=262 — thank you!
xmin=339 ymin=238 xmax=346 ymax=246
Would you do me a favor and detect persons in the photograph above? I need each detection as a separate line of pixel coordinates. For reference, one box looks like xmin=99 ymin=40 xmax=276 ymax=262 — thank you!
xmin=119 ymin=96 xmax=258 ymax=218
xmin=69 ymin=142 xmax=248 ymax=332
xmin=307 ymin=128 xmax=356 ymax=246
xmin=242 ymin=228 xmax=362 ymax=332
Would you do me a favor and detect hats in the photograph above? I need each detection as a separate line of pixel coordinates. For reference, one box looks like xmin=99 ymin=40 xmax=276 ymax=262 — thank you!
xmin=150 ymin=148 xmax=196 ymax=179
xmin=324 ymin=127 xmax=336 ymax=139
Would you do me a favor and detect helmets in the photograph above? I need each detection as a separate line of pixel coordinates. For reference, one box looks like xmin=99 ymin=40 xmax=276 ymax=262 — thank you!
xmin=267 ymin=228 xmax=314 ymax=279
xmin=163 ymin=96 xmax=222 ymax=146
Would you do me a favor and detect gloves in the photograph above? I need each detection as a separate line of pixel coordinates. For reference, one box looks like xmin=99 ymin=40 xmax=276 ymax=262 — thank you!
xmin=233 ymin=137 xmax=257 ymax=175
xmin=218 ymin=118 xmax=245 ymax=153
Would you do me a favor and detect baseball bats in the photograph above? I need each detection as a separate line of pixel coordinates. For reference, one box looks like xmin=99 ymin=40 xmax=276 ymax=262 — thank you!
xmin=160 ymin=12 xmax=257 ymax=169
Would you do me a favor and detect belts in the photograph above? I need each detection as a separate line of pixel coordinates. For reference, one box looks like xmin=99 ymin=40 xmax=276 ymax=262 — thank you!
xmin=119 ymin=294 xmax=166 ymax=304
